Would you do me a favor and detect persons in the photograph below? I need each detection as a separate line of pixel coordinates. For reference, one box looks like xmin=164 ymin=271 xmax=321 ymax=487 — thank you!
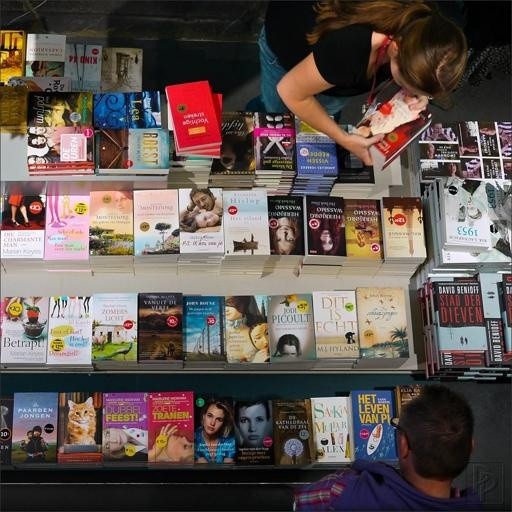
xmin=259 ymin=0 xmax=467 ymax=167
xmin=287 ymin=386 xmax=486 ymax=512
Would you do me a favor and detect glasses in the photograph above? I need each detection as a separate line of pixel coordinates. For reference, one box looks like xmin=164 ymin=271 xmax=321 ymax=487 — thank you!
xmin=387 ymin=415 xmax=413 ymax=455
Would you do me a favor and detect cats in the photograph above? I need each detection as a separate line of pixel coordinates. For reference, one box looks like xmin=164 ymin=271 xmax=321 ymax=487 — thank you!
xmin=67 ymin=396 xmax=96 ymax=447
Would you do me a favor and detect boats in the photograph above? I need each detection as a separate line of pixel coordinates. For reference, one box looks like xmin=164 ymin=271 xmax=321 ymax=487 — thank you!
xmin=233 ymin=241 xmax=258 ymax=246
xmin=234 ymin=246 xmax=258 ymax=252
